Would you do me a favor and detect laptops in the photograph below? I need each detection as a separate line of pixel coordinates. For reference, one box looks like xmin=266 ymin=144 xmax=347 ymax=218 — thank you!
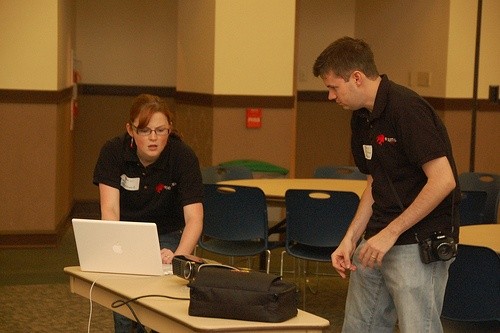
xmin=72 ymin=218 xmax=173 ymax=276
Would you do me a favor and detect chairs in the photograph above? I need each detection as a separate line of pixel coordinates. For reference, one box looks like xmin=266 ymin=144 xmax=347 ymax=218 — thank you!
xmin=193 ymin=166 xmax=368 ymax=312
xmin=439 ymin=172 xmax=500 ymax=333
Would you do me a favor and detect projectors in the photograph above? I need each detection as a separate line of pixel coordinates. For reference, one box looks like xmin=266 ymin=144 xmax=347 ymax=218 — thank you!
xmin=172 ymin=255 xmax=239 ymax=281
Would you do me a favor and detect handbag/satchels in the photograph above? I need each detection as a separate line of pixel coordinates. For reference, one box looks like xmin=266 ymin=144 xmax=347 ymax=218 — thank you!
xmin=188 ymin=263 xmax=298 ymax=323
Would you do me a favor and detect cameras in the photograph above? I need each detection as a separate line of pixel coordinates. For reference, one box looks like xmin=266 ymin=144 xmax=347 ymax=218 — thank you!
xmin=420 ymin=230 xmax=457 ymax=264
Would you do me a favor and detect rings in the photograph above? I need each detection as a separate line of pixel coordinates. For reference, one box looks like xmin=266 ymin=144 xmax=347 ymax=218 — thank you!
xmin=371 ymin=255 xmax=376 ymax=260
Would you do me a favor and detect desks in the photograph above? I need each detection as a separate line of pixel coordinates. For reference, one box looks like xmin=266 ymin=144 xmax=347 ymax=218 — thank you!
xmin=216 ymin=178 xmax=367 ymax=199
xmin=64 ymin=258 xmax=330 ymax=333
xmin=459 ymin=224 xmax=500 ymax=256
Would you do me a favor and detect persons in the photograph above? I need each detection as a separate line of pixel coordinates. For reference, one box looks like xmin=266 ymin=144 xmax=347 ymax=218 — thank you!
xmin=93 ymin=93 xmax=203 ymax=333
xmin=312 ymin=37 xmax=460 ymax=333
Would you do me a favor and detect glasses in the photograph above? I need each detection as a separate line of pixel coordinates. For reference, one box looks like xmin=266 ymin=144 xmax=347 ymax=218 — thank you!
xmin=131 ymin=123 xmax=170 ymax=135
